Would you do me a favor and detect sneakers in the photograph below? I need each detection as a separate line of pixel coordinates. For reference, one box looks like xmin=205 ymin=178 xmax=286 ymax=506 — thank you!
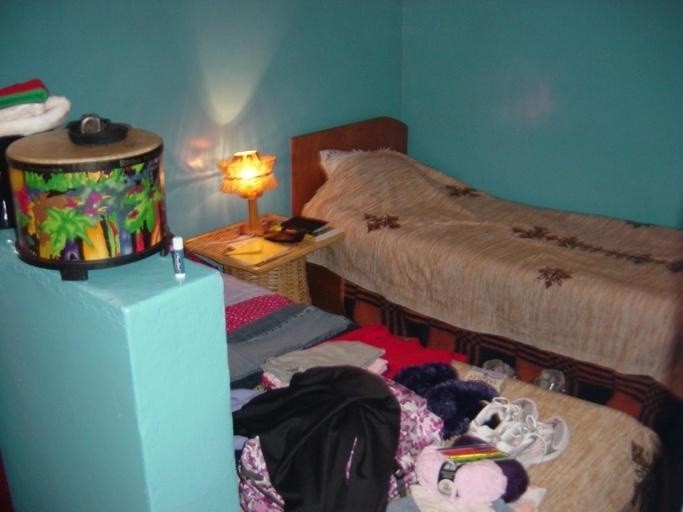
xmin=468 ymin=397 xmax=540 ymax=447
xmin=490 ymin=414 xmax=570 ymax=473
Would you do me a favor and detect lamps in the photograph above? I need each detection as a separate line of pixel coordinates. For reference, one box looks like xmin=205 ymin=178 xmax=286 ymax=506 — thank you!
xmin=216 ymin=147 xmax=279 ymax=233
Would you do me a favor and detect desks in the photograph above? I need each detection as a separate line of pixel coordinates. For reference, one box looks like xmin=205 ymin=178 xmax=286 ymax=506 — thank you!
xmin=181 ymin=214 xmax=348 ymax=309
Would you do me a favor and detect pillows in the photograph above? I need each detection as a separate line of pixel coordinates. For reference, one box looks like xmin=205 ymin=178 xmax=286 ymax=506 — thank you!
xmin=316 ymin=147 xmax=347 ymax=179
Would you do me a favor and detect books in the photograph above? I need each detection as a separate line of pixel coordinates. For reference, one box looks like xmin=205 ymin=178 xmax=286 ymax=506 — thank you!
xmin=280 ymin=215 xmax=331 ymax=235
xmin=280 ymin=223 xmax=337 ymax=242
xmin=226 ymin=236 xmax=290 ymax=264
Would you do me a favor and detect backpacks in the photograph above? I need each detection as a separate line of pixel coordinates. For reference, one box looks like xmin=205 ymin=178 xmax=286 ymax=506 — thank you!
xmin=233 ymin=365 xmax=400 ymax=511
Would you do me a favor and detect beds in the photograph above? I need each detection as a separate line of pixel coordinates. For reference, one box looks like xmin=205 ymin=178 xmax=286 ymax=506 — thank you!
xmin=293 ymin=114 xmax=683 ymax=432
xmin=222 ymin=276 xmax=662 ymax=512
